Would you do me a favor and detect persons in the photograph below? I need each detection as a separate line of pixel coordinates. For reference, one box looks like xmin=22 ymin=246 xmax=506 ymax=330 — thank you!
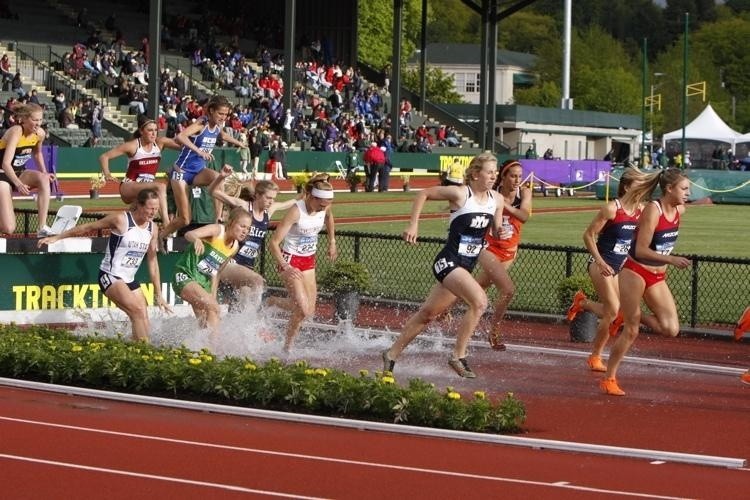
xmin=567 ymin=167 xmax=648 ymax=373
xmin=467 ymin=159 xmax=533 ymax=351
xmin=640 ymin=144 xmax=693 ymax=168
xmin=739 ymin=365 xmax=750 ymax=386
xmin=713 ymin=148 xmax=740 ymax=169
xmin=0 ymin=39 xmax=338 ymax=364
xmin=379 ymin=152 xmax=506 ymax=379
xmin=525 ymin=144 xmax=538 ymax=158
xmin=544 ymin=147 xmax=553 ymax=158
xmin=325 ymin=64 xmax=462 ymax=192
xmin=597 ymin=165 xmax=693 ymax=395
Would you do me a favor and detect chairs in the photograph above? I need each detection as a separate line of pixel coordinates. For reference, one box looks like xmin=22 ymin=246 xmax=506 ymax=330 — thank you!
xmin=334 ymin=160 xmax=347 ymax=180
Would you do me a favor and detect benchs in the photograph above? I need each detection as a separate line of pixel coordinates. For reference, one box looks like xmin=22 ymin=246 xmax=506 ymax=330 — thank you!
xmin=1 ymin=39 xmax=484 ymax=151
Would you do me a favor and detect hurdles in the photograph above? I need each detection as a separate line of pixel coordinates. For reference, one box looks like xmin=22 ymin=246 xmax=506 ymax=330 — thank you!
xmin=0 ymin=237 xmax=247 ymax=327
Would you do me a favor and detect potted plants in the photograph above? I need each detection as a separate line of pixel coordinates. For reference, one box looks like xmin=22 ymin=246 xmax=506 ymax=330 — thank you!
xmin=316 ymin=261 xmax=380 ymax=326
xmin=554 ymin=274 xmax=605 ymax=343
xmin=87 ymin=176 xmax=103 ymax=200
xmin=346 ymin=172 xmax=361 ymax=193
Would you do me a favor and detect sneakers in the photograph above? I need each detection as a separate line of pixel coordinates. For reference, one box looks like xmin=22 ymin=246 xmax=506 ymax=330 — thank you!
xmin=586 ymin=355 xmax=607 ymax=372
xmin=383 ymin=351 xmax=395 ymax=376
xmin=36 ymin=225 xmax=57 ymax=238
xmin=436 ymin=312 xmax=450 ymax=327
xmin=447 ymin=355 xmax=476 ymax=378
xmin=609 ymin=308 xmax=624 ymax=336
xmin=600 ymin=376 xmax=625 ymax=395
xmin=740 ymin=371 xmax=750 ymax=385
xmin=734 ymin=306 xmax=750 ymax=341
xmin=488 ymin=331 xmax=506 ymax=351
xmin=567 ymin=292 xmax=587 ymax=321
